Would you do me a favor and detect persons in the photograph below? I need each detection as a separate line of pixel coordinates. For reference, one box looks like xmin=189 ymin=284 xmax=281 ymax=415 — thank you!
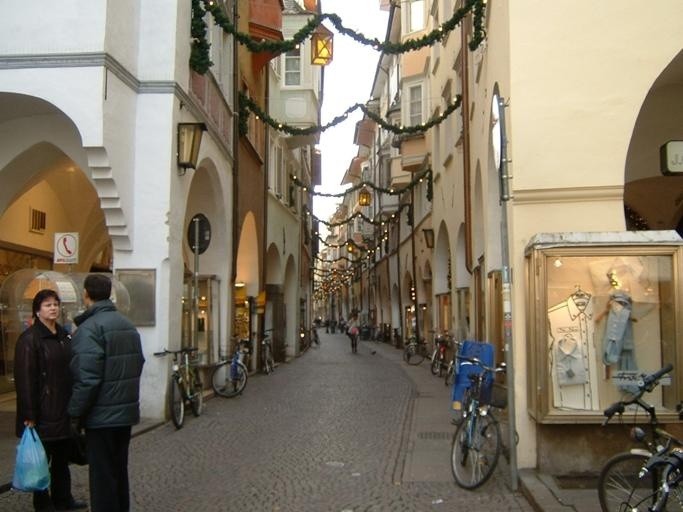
xmin=315 ymin=314 xmax=362 ymax=354
xmin=12 ymin=289 xmax=86 ymax=511
xmin=67 ymin=273 xmax=144 ymax=512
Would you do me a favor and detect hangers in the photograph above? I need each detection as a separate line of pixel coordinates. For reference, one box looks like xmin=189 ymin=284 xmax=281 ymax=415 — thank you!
xmin=594 ymin=297 xmax=638 ymax=324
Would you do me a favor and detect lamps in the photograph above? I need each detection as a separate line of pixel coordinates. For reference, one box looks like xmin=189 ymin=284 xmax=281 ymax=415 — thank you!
xmin=357 ymin=185 xmax=372 ymax=206
xmin=176 ymin=120 xmax=208 ymax=174
xmin=308 ymin=19 xmax=334 ymax=66
xmin=421 ymin=225 xmax=434 ymax=249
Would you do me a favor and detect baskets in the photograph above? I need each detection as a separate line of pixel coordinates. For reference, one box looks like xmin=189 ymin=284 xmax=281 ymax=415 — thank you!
xmin=470 ymin=378 xmax=508 ymax=409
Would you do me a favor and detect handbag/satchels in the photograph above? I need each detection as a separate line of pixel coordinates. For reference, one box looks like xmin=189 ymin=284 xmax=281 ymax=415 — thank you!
xmin=55 ymin=430 xmax=91 ymax=465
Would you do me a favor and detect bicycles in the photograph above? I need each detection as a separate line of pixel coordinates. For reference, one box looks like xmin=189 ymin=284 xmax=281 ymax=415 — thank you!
xmin=440 ymin=336 xmax=464 ymax=384
xmin=152 ymin=345 xmax=206 ymax=430
xmin=446 ymin=339 xmax=506 ymax=495
xmin=358 ymin=323 xmax=390 ymax=343
xmin=309 ymin=324 xmax=321 ymax=349
xmin=208 ymin=339 xmax=255 ymax=400
xmin=594 ymin=363 xmax=682 ymax=512
xmin=429 ymin=327 xmax=459 ymax=376
xmin=632 ymin=438 xmax=683 ymax=512
xmin=400 ymin=334 xmax=428 ymax=366
xmin=257 ymin=327 xmax=277 ymax=376
